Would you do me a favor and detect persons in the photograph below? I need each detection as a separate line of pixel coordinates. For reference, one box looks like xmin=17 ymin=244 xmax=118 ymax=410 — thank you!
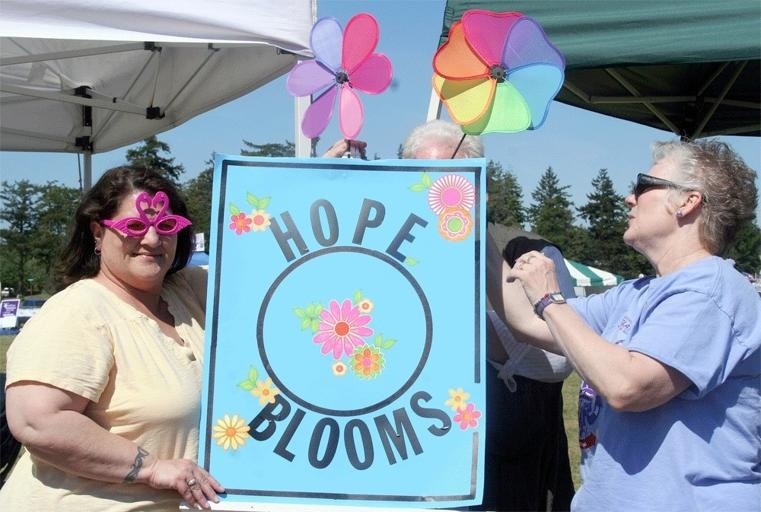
xmin=0 ymin=139 xmax=363 ymax=512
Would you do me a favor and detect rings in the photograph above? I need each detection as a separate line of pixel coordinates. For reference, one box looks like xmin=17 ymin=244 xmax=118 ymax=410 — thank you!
xmin=187 ymin=479 xmax=196 ymax=486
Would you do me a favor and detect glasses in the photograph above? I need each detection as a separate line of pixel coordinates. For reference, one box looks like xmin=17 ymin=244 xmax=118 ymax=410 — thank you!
xmin=634 ymin=173 xmax=706 ymax=203
